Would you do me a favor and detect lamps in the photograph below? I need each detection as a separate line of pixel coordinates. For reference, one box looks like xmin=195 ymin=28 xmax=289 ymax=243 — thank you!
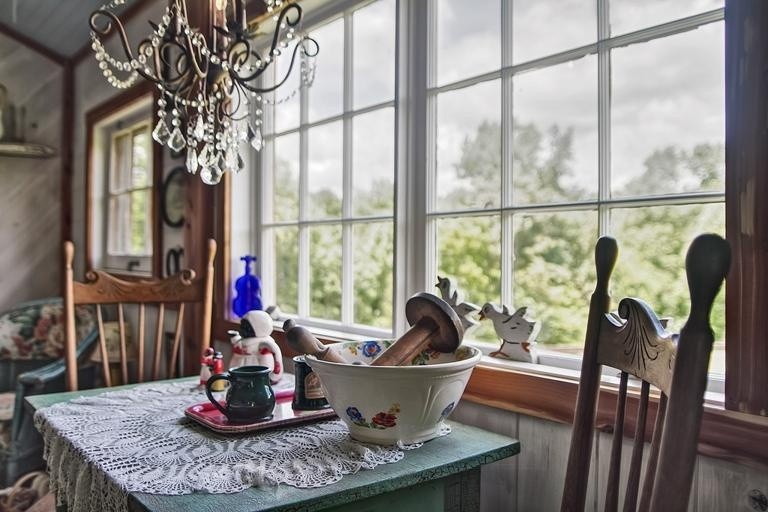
xmin=87 ymin=0 xmax=319 ymax=186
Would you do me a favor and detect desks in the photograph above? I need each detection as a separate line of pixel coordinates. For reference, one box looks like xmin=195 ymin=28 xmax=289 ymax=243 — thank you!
xmin=25 ymin=371 xmax=521 ymax=512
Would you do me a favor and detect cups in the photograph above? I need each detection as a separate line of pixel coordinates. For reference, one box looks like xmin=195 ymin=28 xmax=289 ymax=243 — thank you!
xmin=206 ymin=365 xmax=275 ymax=424
xmin=229 ymin=335 xmax=283 ymax=384
xmin=291 ymin=355 xmax=332 ymax=410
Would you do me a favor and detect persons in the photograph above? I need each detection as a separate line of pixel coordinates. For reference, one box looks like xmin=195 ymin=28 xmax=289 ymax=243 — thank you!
xmin=227 ymin=309 xmax=286 ymax=384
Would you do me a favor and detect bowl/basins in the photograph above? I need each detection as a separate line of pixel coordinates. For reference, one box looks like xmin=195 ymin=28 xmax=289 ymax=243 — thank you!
xmin=304 ymin=339 xmax=482 ymax=447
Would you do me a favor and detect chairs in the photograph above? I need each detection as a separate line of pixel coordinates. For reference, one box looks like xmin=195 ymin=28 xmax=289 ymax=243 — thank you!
xmin=0 ymin=300 xmax=106 ymax=501
xmin=558 ymin=233 xmax=731 ymax=511
xmin=63 ymin=235 xmax=221 ymax=396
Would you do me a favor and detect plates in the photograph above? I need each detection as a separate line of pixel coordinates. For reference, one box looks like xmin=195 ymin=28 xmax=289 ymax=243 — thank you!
xmin=186 ymin=387 xmax=335 ymax=435
